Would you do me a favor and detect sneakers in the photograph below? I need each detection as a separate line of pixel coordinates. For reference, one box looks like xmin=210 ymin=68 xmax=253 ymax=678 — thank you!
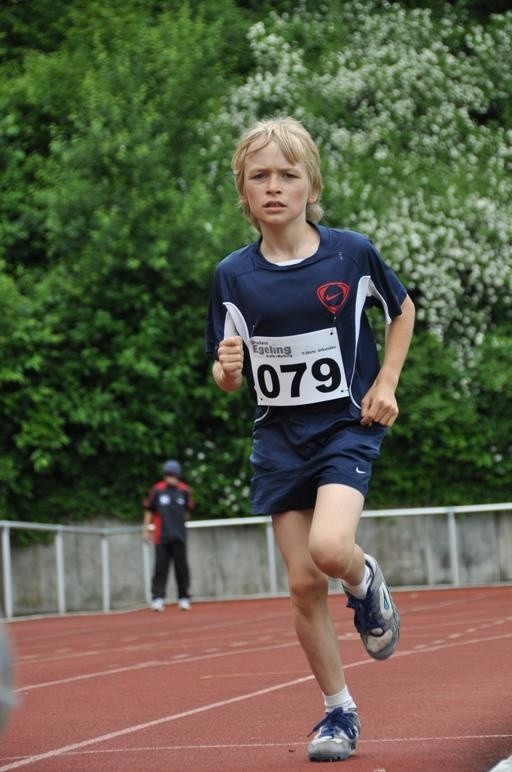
xmin=151 ymin=597 xmax=164 ymax=611
xmin=341 ymin=553 xmax=400 ymax=660
xmin=179 ymin=597 xmax=191 ymax=610
xmin=308 ymin=705 xmax=361 ymax=761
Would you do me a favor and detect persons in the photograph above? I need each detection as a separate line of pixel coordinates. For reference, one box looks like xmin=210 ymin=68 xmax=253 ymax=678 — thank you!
xmin=205 ymin=115 xmax=416 ymax=760
xmin=143 ymin=460 xmax=195 ymax=612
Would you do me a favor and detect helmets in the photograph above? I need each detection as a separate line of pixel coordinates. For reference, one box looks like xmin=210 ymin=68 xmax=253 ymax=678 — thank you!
xmin=163 ymin=459 xmax=180 ymax=476
